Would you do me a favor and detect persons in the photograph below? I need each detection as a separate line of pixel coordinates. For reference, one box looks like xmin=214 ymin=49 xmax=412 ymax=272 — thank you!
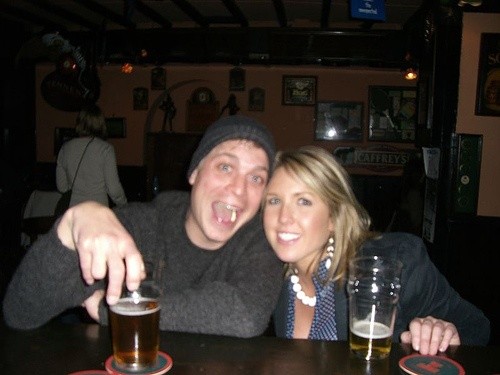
xmin=262 ymin=145 xmax=491 ymax=357
xmin=2 ymin=116 xmax=282 ymax=339
xmin=56 ymin=103 xmax=129 ymax=210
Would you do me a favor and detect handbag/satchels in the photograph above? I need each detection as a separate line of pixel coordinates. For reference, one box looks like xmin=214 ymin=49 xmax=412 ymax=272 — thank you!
xmin=55 ymin=189 xmax=72 ymax=218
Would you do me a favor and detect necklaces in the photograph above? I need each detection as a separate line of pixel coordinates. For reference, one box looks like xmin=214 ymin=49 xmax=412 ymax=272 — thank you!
xmin=290 ymin=271 xmax=317 ymax=307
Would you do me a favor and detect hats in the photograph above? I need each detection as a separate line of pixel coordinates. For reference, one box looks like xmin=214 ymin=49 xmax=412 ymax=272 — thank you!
xmin=186 ymin=114 xmax=277 ymax=182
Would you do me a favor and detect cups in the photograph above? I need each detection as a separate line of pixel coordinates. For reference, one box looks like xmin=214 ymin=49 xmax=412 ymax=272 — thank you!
xmin=347 ymin=255 xmax=404 ymax=362
xmin=106 ymin=261 xmax=163 ymax=374
